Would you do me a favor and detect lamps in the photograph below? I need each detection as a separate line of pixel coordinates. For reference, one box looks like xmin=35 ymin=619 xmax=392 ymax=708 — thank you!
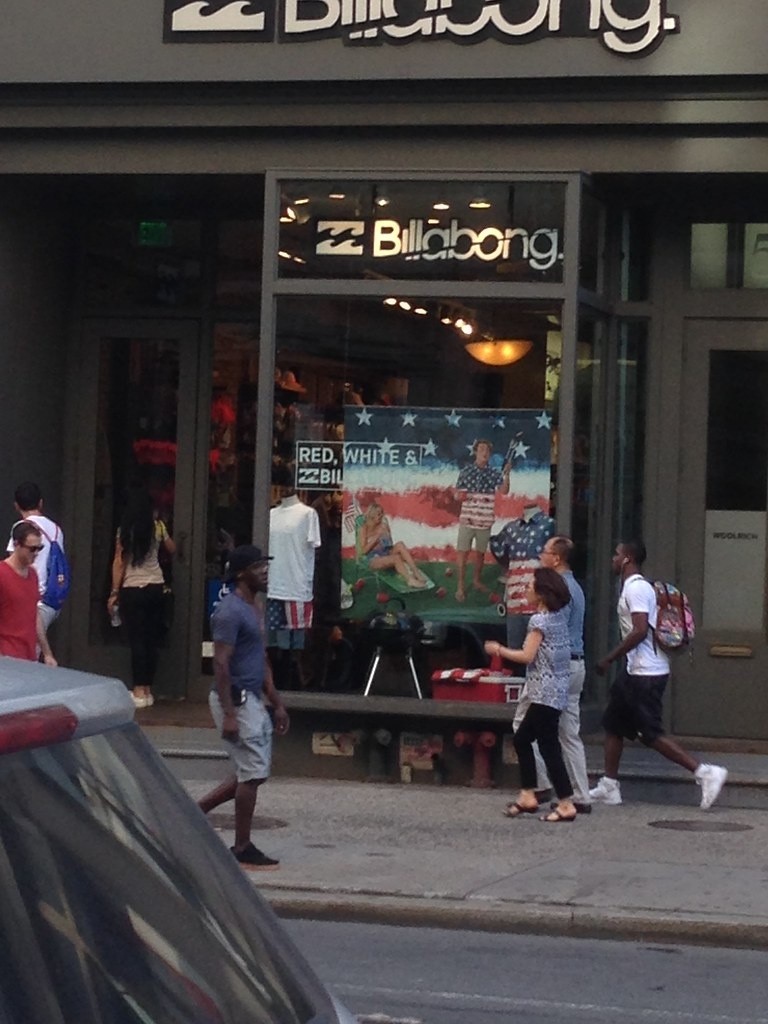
xmin=465 ymin=340 xmax=535 ymax=368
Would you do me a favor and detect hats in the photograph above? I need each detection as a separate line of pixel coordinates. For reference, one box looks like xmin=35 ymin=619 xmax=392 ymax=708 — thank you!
xmin=228 ymin=544 xmax=274 ymax=576
xmin=270 ymin=465 xmax=294 ymax=486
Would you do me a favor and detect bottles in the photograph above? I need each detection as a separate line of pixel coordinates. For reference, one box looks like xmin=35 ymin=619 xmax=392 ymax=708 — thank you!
xmin=110 ymin=601 xmax=122 ymax=627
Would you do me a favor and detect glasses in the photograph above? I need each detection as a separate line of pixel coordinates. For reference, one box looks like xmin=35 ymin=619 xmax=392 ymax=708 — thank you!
xmin=20 ymin=544 xmax=44 ymax=553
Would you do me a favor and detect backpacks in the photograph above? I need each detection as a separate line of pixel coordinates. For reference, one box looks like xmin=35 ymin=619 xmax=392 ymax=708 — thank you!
xmin=625 ymin=577 xmax=695 ymax=654
xmin=26 ymin=519 xmax=70 ymax=611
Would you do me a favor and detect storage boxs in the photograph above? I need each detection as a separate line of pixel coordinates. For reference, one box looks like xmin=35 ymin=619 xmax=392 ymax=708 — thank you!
xmin=431 ymin=676 xmax=526 ymax=705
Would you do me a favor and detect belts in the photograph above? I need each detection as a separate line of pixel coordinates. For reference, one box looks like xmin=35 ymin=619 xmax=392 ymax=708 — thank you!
xmin=571 ymin=653 xmax=586 ymax=660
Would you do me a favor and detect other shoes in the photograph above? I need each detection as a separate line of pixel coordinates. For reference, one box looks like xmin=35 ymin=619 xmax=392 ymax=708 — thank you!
xmin=229 ymin=842 xmax=279 ymax=871
xmin=128 ymin=690 xmax=146 ymax=708
xmin=145 ymin=694 xmax=154 ymax=706
xmin=549 ymin=802 xmax=593 ymax=814
xmin=505 ymin=788 xmax=555 ymax=806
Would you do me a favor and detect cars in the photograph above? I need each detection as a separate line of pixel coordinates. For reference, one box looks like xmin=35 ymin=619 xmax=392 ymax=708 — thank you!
xmin=0 ymin=651 xmax=362 ymax=1023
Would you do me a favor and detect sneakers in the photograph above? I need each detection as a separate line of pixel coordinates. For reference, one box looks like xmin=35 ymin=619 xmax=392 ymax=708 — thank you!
xmin=570 ymin=776 xmax=623 ymax=805
xmin=695 ymin=764 xmax=728 ymax=810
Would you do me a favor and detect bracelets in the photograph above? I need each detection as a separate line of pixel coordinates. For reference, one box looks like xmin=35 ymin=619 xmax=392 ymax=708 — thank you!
xmin=496 ymin=646 xmax=501 ymax=656
xmin=111 ymin=589 xmax=118 ymax=595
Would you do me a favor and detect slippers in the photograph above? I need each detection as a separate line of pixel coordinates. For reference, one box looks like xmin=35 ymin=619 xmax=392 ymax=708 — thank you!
xmin=537 ymin=809 xmax=576 ymax=822
xmin=500 ymin=800 xmax=538 ymax=819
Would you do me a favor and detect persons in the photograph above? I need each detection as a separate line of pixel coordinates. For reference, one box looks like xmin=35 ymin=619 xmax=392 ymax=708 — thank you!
xmin=0 ymin=522 xmax=58 ymax=667
xmin=485 ymin=567 xmax=577 ymax=822
xmin=503 ymin=504 xmax=556 ymax=678
xmin=107 ymin=491 xmax=177 ymax=708
xmin=196 ymin=544 xmax=290 ymax=870
xmin=268 ymin=495 xmax=323 ymax=631
xmin=6 ymin=481 xmax=67 ymax=660
xmin=590 ymin=536 xmax=728 ymax=811
xmin=512 ymin=535 xmax=592 ymax=813
xmin=454 ymin=440 xmax=512 ymax=602
xmin=361 ymin=503 xmax=429 ymax=588
